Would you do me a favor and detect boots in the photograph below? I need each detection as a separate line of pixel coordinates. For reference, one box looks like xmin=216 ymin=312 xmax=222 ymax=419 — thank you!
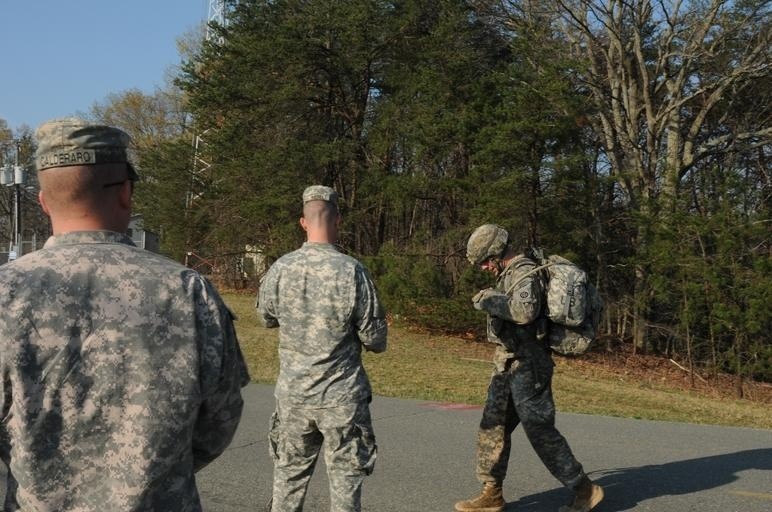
xmin=455 ymin=484 xmax=505 ymax=512
xmin=558 ymin=484 xmax=604 ymax=512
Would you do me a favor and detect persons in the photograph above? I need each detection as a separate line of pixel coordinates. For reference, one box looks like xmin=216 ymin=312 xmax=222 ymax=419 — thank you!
xmin=256 ymin=185 xmax=389 ymax=512
xmin=453 ymin=223 xmax=605 ymax=512
xmin=0 ymin=118 xmax=249 ymax=512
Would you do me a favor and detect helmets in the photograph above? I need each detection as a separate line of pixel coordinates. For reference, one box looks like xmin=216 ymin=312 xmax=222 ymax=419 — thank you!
xmin=466 ymin=223 xmax=509 ymax=266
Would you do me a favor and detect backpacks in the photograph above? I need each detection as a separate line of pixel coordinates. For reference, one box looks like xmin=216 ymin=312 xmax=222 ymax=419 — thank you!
xmin=504 ymin=254 xmax=604 ymax=356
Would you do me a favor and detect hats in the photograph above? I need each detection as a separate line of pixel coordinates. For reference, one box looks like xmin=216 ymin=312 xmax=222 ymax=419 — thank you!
xmin=303 ymin=185 xmax=338 ymax=206
xmin=35 ymin=119 xmax=140 ymax=181
